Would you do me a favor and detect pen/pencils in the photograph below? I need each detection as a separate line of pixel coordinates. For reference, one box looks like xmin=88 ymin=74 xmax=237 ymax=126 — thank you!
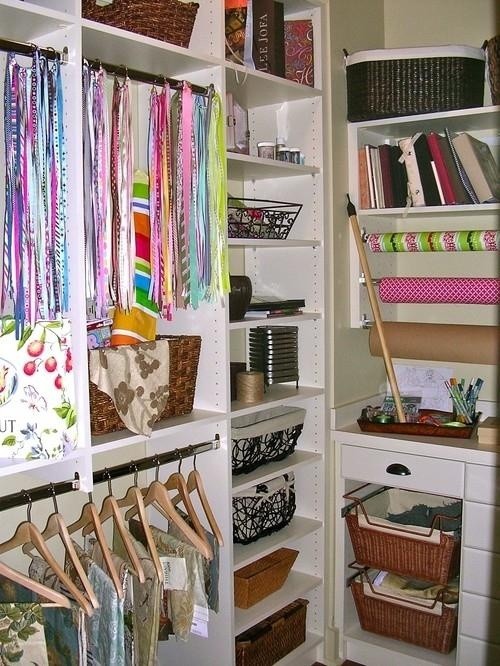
xmin=444 ymin=377 xmax=484 ymax=425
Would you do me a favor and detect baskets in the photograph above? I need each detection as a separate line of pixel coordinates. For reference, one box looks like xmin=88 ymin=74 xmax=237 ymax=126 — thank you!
xmin=342 ymin=34 xmax=500 ymax=122
xmin=88 ymin=335 xmax=201 ymax=436
xmin=82 ymin=0 xmax=200 ymax=49
xmin=341 ymin=483 xmax=462 ymax=655
xmin=227 ymin=197 xmax=303 ymax=239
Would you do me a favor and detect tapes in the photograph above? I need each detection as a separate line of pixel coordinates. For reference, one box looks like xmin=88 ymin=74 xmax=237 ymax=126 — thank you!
xmin=375 ymin=415 xmax=392 ymax=423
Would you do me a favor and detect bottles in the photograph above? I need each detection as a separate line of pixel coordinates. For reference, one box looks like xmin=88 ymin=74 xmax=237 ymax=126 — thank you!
xmin=257 ymin=137 xmax=301 ymax=164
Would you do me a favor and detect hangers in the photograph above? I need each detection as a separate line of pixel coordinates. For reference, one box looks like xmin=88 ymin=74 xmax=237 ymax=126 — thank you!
xmin=0 ymin=434 xmax=228 ymax=618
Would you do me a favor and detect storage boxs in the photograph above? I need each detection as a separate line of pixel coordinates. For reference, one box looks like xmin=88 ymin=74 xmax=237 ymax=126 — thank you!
xmin=342 ymin=41 xmax=485 ymax=117
xmin=342 ymin=481 xmax=466 ymax=586
xmin=345 ymin=561 xmax=454 ymax=655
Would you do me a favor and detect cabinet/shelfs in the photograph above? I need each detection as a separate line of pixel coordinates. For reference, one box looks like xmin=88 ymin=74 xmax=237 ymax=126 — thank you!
xmin=78 ymin=0 xmax=230 ymax=448
xmin=225 ymin=391 xmax=334 ymax=664
xmin=5 ymin=5 xmax=87 ymax=466
xmin=221 ymin=0 xmax=337 ymax=418
xmin=341 ymin=105 xmax=498 ymax=453
xmin=0 ymin=415 xmax=231 ymax=666
xmin=329 ymin=430 xmax=498 ymax=666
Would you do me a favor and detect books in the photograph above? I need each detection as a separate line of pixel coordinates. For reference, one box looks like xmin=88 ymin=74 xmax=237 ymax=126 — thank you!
xmin=359 ymin=128 xmax=500 ymax=208
